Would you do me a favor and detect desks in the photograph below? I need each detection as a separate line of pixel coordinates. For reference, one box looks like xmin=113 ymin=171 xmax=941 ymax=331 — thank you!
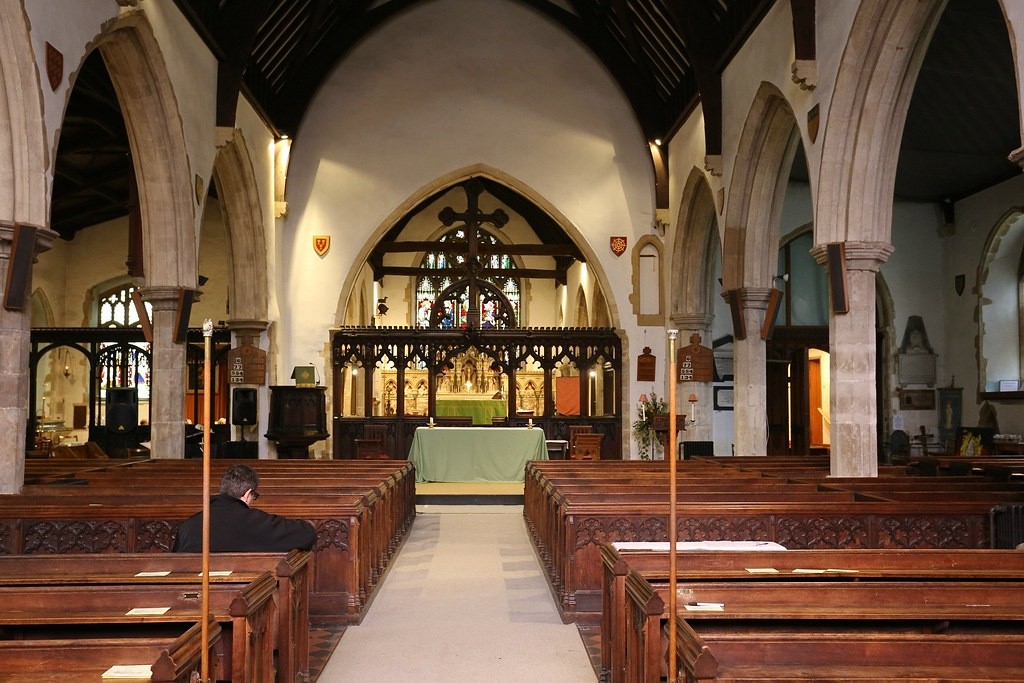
xmin=575 ymin=433 xmax=603 ymax=460
xmin=407 ymin=426 xmax=549 ymax=496
xmin=546 ymin=440 xmax=568 ymax=460
xmin=436 ymin=399 xmax=507 ymax=425
xmin=354 ymin=439 xmax=381 ymax=460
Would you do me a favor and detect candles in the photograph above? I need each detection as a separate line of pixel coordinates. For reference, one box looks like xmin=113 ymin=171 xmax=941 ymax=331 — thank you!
xmin=529 ymin=419 xmax=532 ymax=425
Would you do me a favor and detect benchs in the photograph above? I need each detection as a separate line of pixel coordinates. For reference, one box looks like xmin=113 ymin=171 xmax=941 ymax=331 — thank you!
xmin=0 ymin=457 xmax=416 ymax=683
xmin=523 ymin=454 xmax=1024 ymax=683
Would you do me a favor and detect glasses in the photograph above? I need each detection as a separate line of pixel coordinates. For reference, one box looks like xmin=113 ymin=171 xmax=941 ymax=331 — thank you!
xmin=241 ymin=489 xmax=260 ymax=500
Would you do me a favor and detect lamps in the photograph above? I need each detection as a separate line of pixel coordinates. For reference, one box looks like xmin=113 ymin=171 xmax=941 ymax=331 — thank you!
xmin=278 ymin=128 xmax=288 ymax=139
xmin=688 ymin=394 xmax=698 ymax=422
xmin=772 ymin=273 xmax=788 ymax=281
xmin=640 ymin=394 xmax=647 ymax=421
xmin=64 ymin=366 xmax=70 ymax=378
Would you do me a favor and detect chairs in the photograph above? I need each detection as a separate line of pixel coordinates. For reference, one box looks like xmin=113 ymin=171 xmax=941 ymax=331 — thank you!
xmin=363 ymin=425 xmax=388 ymax=459
xmin=569 ymin=425 xmax=592 ymax=459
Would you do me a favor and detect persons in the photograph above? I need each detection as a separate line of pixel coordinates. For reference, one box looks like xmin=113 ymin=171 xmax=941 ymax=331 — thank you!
xmin=171 ymin=465 xmax=318 ymax=552
xmin=218 ymin=418 xmax=226 ymax=424
xmin=140 ymin=418 xmax=147 ymax=425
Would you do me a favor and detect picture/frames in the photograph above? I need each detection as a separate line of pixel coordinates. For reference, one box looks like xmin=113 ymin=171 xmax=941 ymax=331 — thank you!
xmin=186 ymin=360 xmax=219 ymax=395
